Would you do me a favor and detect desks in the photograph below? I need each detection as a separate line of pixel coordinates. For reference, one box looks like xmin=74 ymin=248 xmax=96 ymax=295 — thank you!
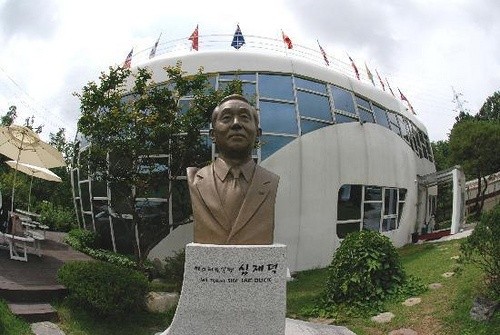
xmin=8 ymin=212 xmax=32 ymax=229
xmin=15 ymin=209 xmax=41 ymax=221
xmin=0 ymin=232 xmax=35 ymax=262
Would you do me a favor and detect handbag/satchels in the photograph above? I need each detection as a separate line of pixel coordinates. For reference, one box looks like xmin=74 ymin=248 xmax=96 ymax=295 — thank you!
xmin=0 ymin=207 xmax=24 ymax=237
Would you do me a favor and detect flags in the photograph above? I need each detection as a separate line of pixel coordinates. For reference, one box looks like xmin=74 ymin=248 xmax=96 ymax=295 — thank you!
xmin=281 ymin=28 xmax=293 ymax=49
xmin=188 ymin=24 xmax=198 ymax=51
xmin=317 ymin=40 xmax=330 ymax=66
xmin=348 ymin=56 xmax=416 ymax=115
xmin=231 ymin=22 xmax=246 ymax=50
xmin=149 ymin=33 xmax=162 ymax=60
xmin=121 ymin=48 xmax=133 ymax=70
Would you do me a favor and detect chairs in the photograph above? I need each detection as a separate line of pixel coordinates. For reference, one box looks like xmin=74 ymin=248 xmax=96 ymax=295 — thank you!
xmin=0 ymin=208 xmax=49 ymax=264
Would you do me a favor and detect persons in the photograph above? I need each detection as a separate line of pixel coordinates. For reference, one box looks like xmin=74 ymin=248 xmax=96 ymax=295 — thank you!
xmin=186 ymin=94 xmax=280 ymax=246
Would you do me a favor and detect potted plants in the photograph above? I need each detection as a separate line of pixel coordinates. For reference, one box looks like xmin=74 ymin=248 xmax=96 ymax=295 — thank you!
xmin=411 ymin=220 xmax=419 ymax=243
